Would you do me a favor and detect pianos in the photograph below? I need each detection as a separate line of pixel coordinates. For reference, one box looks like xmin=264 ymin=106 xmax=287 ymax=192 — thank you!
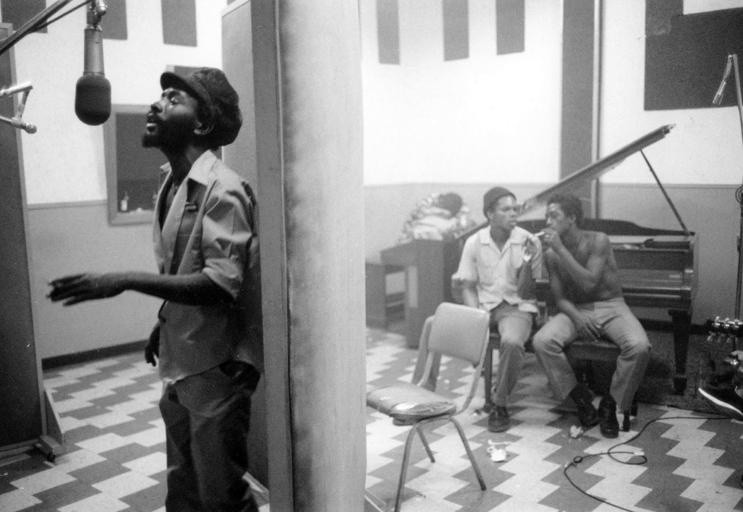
xmin=447 ymin=124 xmax=698 ymax=395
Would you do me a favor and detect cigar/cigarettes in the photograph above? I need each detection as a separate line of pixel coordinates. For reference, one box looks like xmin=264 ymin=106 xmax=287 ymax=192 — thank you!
xmin=535 ymin=231 xmax=545 ymax=237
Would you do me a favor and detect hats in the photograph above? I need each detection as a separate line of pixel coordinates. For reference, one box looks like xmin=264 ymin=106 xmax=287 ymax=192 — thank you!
xmin=484 ymin=186 xmax=516 ymax=217
xmin=160 ymin=67 xmax=242 ymax=145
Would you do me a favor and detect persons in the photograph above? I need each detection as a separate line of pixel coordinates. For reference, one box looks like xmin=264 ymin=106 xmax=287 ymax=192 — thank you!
xmin=532 ymin=192 xmax=655 ymax=440
xmin=44 ymin=68 xmax=264 ymax=512
xmin=389 ymin=185 xmax=547 ymax=432
xmin=698 ymin=349 xmax=743 ymax=418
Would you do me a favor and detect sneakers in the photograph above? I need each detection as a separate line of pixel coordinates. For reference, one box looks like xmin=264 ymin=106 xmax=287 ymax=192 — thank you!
xmin=698 ymin=387 xmax=742 ymax=417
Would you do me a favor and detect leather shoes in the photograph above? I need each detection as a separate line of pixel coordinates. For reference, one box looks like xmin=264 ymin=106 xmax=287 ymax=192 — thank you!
xmin=488 ymin=406 xmax=510 ymax=432
xmin=574 ymin=390 xmax=619 ymax=438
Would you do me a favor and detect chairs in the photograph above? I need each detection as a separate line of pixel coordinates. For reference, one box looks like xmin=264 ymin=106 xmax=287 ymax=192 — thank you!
xmin=364 ymin=301 xmax=493 ymax=512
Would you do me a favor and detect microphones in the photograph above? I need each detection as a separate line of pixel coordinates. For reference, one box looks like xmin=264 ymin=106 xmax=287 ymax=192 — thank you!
xmin=712 ymin=55 xmax=734 ymax=105
xmin=74 ymin=3 xmax=112 ymax=127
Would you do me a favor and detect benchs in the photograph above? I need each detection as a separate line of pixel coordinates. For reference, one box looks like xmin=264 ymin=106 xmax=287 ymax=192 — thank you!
xmin=486 ymin=327 xmax=637 ymax=431
